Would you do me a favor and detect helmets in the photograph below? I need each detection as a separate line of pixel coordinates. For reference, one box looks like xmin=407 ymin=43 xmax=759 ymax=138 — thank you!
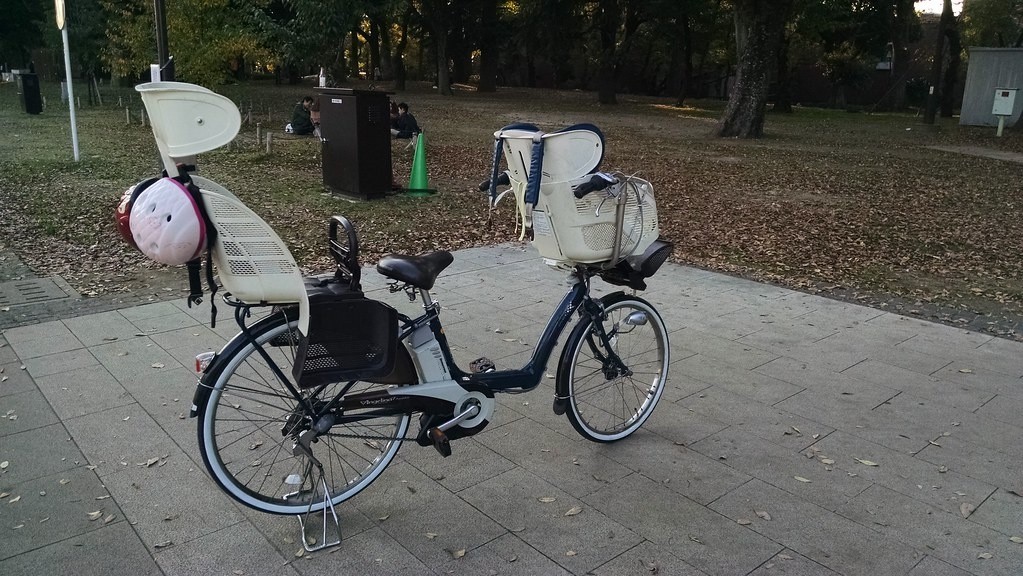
xmin=129 ymin=177 xmax=206 ymax=265
xmin=285 ymin=124 xmax=294 ymax=134
xmin=115 ymin=178 xmax=160 ymax=254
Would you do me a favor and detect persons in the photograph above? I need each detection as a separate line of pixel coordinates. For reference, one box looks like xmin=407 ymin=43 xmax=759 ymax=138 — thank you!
xmin=291 ymin=94 xmax=422 ymax=139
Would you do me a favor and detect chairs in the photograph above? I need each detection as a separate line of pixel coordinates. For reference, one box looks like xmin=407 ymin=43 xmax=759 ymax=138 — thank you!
xmin=494 ymin=122 xmax=659 ymax=265
xmin=134 ymin=82 xmax=398 ymax=390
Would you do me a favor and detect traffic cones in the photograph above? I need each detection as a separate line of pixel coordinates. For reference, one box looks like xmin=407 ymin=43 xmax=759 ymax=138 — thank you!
xmin=402 ymin=131 xmax=438 ymax=196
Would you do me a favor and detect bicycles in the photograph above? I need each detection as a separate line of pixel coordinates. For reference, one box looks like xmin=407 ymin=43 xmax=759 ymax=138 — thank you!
xmin=191 ymin=169 xmax=674 ymax=553
